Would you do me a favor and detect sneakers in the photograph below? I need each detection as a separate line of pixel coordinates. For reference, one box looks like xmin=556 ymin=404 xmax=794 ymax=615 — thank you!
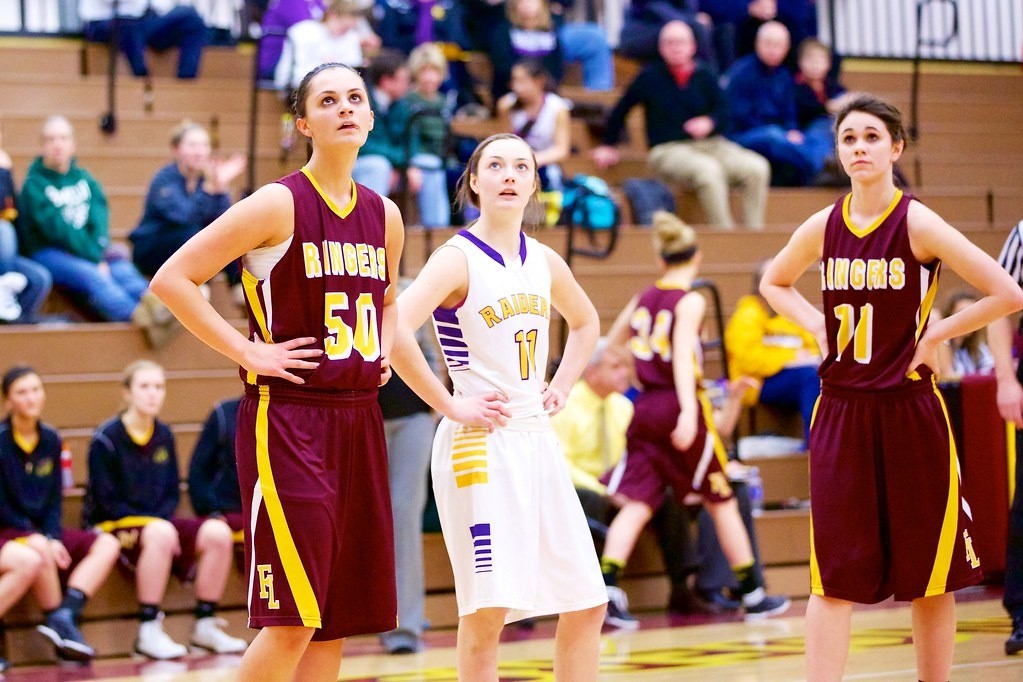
xmin=134 ymin=611 xmax=188 ymax=659
xmin=742 ymin=587 xmax=793 ymax=619
xmin=604 ymin=586 xmax=641 ymax=629
xmin=192 ymin=617 xmax=249 ymax=654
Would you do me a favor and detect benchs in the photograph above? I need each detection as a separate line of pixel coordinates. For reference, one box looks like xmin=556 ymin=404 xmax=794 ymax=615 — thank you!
xmin=0 ymin=30 xmax=1023 ymax=665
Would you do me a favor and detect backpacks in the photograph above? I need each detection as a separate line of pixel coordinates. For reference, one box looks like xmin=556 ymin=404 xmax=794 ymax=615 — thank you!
xmin=561 ymin=175 xmax=616 ymax=230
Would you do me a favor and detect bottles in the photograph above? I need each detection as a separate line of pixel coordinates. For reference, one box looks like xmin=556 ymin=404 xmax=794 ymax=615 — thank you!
xmin=744 ymin=466 xmax=766 ymax=518
xmin=59 ymin=442 xmax=75 ymax=487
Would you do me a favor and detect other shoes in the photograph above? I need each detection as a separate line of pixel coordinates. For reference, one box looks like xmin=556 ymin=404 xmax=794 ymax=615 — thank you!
xmin=0 ymin=273 xmax=28 ymax=320
xmin=1005 ymin=624 xmax=1023 ymax=655
xmin=665 ymin=593 xmax=723 ymax=615
xmin=143 ymin=304 xmax=185 ymax=353
xmin=694 ymin=588 xmax=739 ymax=611
xmin=383 ymin=632 xmax=418 ymax=655
xmin=36 ymin=608 xmax=96 ymax=663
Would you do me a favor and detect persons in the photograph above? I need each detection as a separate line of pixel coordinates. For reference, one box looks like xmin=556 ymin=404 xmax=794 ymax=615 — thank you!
xmin=148 ymin=63 xmax=405 ymax=682
xmin=0 ymin=0 xmax=1023 ymax=682
